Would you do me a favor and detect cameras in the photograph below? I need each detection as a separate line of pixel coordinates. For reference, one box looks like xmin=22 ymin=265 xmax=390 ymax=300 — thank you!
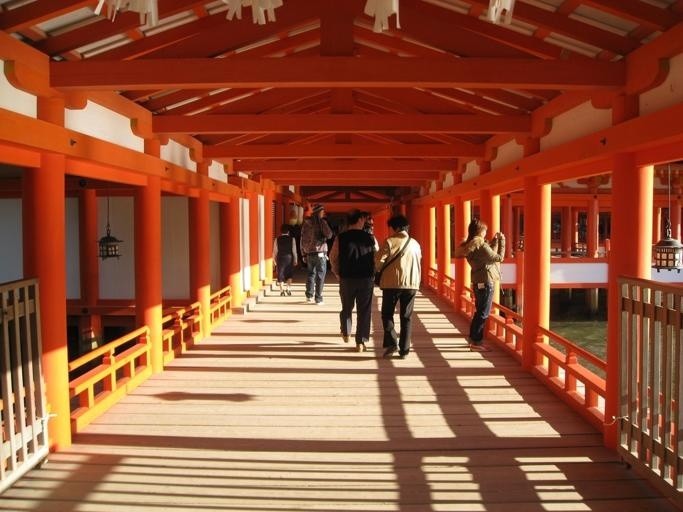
xmin=497 ymin=232 xmax=502 ymax=240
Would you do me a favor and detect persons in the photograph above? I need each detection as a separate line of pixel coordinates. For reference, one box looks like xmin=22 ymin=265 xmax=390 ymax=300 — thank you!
xmin=272 ymin=203 xmax=374 ymax=305
xmin=455 ymin=216 xmax=506 ymax=352
xmin=373 ymin=212 xmax=423 ymax=359
xmin=329 ymin=208 xmax=380 ymax=352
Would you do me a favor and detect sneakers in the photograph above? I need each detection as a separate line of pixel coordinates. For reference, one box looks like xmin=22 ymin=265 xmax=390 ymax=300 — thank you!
xmin=306 ymin=296 xmax=323 ymax=303
xmin=280 ymin=289 xmax=291 ymax=296
xmin=383 ymin=345 xmax=406 ymax=359
xmin=467 ymin=340 xmax=493 ymax=352
xmin=356 ymin=341 xmax=366 ymax=352
xmin=340 ymin=331 xmax=350 ymax=340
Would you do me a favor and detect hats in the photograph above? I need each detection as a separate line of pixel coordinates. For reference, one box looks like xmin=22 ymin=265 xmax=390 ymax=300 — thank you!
xmin=387 ymin=215 xmax=407 ymax=227
xmin=312 ymin=203 xmax=326 ymax=212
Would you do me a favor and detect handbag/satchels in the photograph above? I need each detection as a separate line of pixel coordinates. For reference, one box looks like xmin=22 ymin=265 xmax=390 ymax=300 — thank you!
xmin=375 ymin=270 xmax=382 ymax=284
xmin=319 ymin=231 xmax=327 ymax=242
